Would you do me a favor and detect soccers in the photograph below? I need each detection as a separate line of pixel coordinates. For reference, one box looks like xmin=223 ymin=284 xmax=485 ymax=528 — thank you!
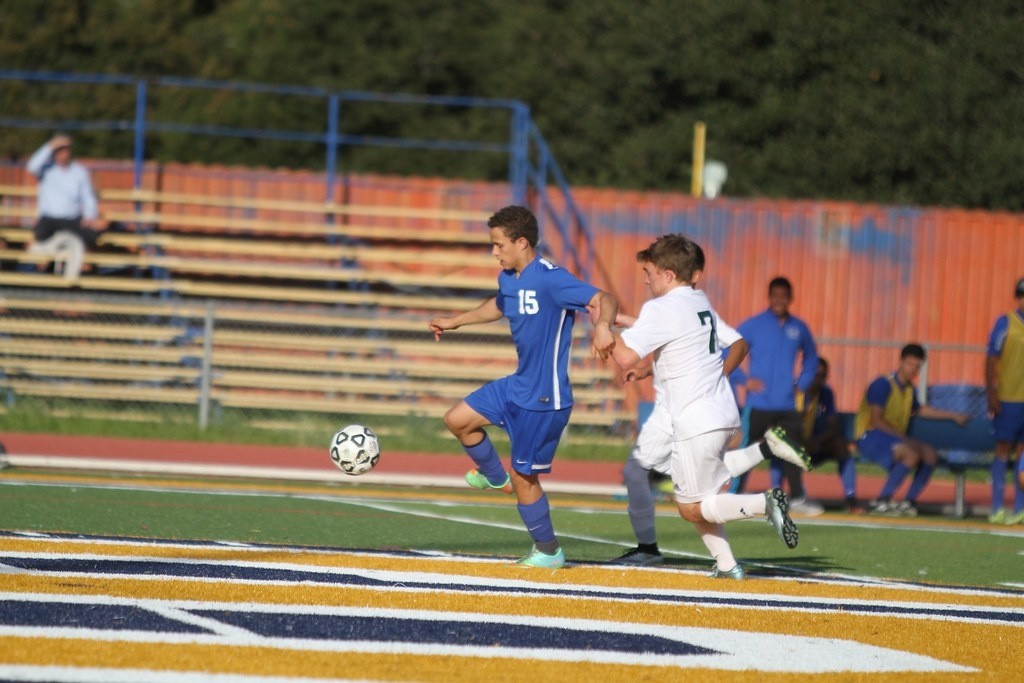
xmin=329 ymin=425 xmax=381 ymax=476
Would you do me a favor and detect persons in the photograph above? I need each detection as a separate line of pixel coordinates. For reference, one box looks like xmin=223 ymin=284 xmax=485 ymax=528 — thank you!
xmin=585 ymin=235 xmax=812 ymax=581
xmin=27 ymin=133 xmax=108 ymax=275
xmin=721 ymin=276 xmax=825 ymax=519
xmin=983 ymin=279 xmax=1024 ymax=524
xmin=818 ymin=343 xmax=978 ymax=516
xmin=427 ymin=205 xmax=620 ymax=569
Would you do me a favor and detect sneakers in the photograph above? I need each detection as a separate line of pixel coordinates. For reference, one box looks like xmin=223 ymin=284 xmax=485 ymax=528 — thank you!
xmin=865 ymin=501 xmax=903 ymax=517
xmin=893 ymin=500 xmax=916 ymax=518
xmin=502 ymin=544 xmax=566 ymax=569
xmin=463 ymin=467 xmax=514 ymax=495
xmin=608 ymin=543 xmax=662 ymax=566
xmin=762 ymin=426 xmax=813 ymax=473
xmin=708 ymin=562 xmax=746 ymax=580
xmin=763 ymin=488 xmax=799 ymax=548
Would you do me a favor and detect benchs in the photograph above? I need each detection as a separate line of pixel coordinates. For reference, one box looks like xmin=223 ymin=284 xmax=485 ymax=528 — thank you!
xmin=0 ymin=185 xmax=640 ymax=433
xmin=836 ymin=412 xmax=997 ymax=518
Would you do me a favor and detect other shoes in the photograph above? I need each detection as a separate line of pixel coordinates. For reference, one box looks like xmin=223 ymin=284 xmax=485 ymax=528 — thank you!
xmin=989 ymin=508 xmax=1024 ymax=526
xmin=845 ymin=498 xmax=867 ymax=515
xmin=785 ymin=498 xmax=826 ymax=515
